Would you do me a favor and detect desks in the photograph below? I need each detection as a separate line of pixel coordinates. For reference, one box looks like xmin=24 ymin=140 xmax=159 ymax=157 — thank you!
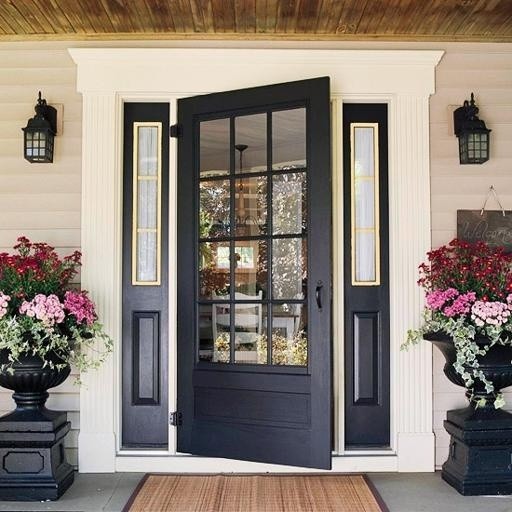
xmin=195 ymin=314 xmax=300 ymax=364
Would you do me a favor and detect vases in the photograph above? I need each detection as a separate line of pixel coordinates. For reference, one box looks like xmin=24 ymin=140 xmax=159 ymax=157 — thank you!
xmin=0 ymin=350 xmax=76 ymax=501
xmin=422 ymin=333 xmax=512 ymax=496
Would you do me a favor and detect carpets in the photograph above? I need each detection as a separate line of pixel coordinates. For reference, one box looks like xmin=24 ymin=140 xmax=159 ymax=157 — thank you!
xmin=121 ymin=471 xmax=390 ymax=511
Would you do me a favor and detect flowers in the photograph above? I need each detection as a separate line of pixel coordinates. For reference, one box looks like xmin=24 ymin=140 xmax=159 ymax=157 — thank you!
xmin=401 ymin=239 xmax=512 ymax=409
xmin=0 ymin=235 xmax=114 ymax=388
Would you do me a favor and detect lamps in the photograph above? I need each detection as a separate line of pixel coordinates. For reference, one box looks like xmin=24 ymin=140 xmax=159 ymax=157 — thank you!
xmin=446 ymin=92 xmax=493 ymax=165
xmin=217 ymin=143 xmax=265 ymax=238
xmin=22 ymin=91 xmax=65 ymax=165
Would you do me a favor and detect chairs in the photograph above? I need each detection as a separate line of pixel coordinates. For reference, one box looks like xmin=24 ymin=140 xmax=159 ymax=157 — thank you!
xmin=211 ymin=290 xmax=264 ymax=363
